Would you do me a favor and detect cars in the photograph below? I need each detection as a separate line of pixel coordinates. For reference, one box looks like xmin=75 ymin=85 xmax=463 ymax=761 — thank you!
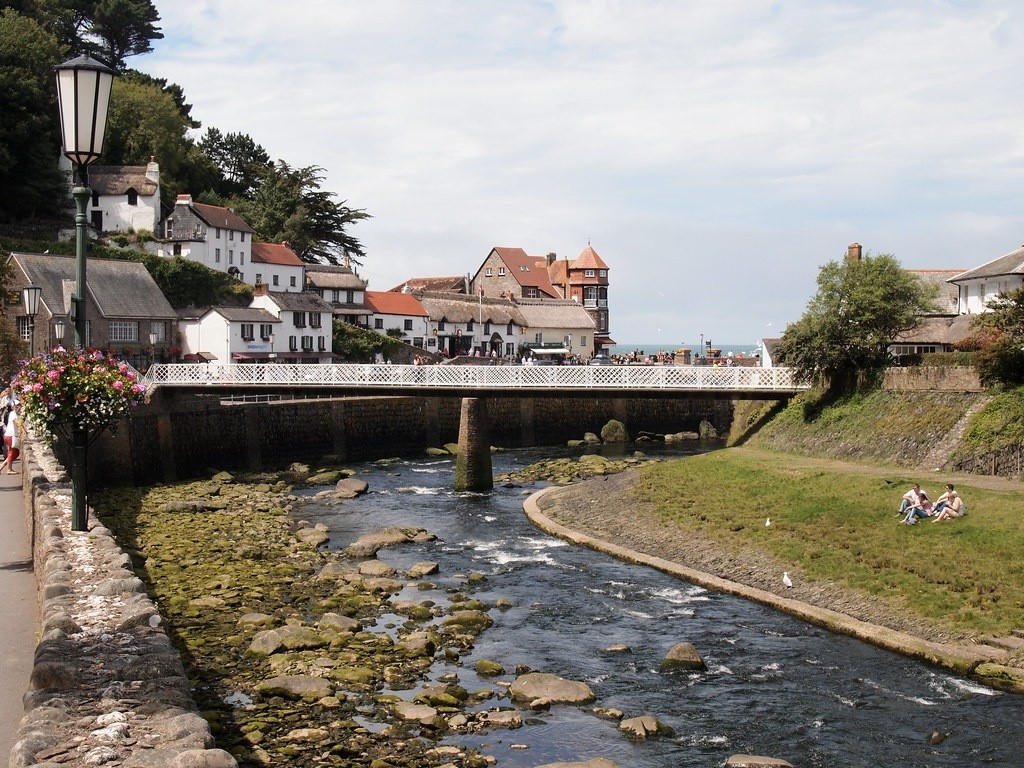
xmin=589 ymin=355 xmax=614 ymax=365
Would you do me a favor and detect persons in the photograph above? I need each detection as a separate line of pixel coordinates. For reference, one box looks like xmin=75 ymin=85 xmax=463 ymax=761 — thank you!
xmin=1 ymin=389 xmax=23 ymax=476
xmin=413 ymin=345 xmax=762 ymax=368
xmin=931 ymin=491 xmax=965 ymax=523
xmin=893 ymin=484 xmax=930 ymax=517
xmin=899 ymin=493 xmax=932 ymax=525
xmin=931 ymin=484 xmax=957 ymax=517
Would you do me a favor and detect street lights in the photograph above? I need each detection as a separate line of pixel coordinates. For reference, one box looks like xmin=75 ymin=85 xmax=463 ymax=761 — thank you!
xmin=53 ymin=51 xmax=121 ymax=529
xmin=55 ymin=321 xmax=66 ymax=351
xmin=21 ymin=281 xmax=41 ymax=358
xmin=149 ymin=332 xmax=158 ymax=365
xmin=699 ymin=333 xmax=704 ymax=357
xmin=269 ymin=333 xmax=277 ymax=364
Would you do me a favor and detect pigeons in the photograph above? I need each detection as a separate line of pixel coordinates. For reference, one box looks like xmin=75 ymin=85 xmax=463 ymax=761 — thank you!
xmin=782 ymin=571 xmax=792 ymax=589
xmin=764 ymin=518 xmax=771 ymax=529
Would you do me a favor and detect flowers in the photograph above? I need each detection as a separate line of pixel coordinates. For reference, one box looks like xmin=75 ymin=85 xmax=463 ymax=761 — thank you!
xmin=11 ymin=348 xmax=149 ymax=441
